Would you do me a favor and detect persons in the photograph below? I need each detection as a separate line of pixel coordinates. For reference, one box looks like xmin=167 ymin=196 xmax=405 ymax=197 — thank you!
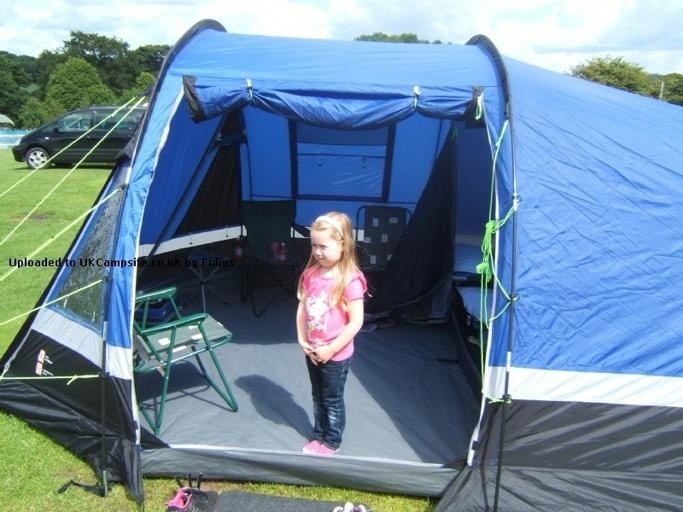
xmin=295 ymin=211 xmax=368 ymax=456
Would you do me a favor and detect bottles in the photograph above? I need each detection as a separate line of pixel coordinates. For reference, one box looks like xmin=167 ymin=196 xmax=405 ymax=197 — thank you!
xmin=278 ymin=243 xmax=288 ymax=262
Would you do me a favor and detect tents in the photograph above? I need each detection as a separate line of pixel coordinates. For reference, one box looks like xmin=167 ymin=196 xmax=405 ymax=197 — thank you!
xmin=0 ymin=19 xmax=683 ymax=512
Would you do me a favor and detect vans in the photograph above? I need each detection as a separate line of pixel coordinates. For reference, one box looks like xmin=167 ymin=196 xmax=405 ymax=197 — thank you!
xmin=12 ymin=106 xmax=148 ymax=169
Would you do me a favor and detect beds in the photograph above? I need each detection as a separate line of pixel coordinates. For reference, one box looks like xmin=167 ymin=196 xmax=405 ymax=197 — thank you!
xmin=450 ymin=270 xmax=498 ymax=400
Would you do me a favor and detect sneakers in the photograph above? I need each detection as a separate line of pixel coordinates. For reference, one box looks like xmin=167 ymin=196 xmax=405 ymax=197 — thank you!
xmin=303 ymin=440 xmax=340 ymax=455
xmin=166 ymin=488 xmax=218 ymax=512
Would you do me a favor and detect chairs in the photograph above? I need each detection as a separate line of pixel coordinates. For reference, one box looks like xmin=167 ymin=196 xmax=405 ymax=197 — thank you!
xmin=133 ymin=267 xmax=180 ymax=329
xmin=132 ymin=287 xmax=238 ymax=436
xmin=355 ymin=205 xmax=411 ymax=271
xmin=240 ymin=199 xmax=311 ymax=317
